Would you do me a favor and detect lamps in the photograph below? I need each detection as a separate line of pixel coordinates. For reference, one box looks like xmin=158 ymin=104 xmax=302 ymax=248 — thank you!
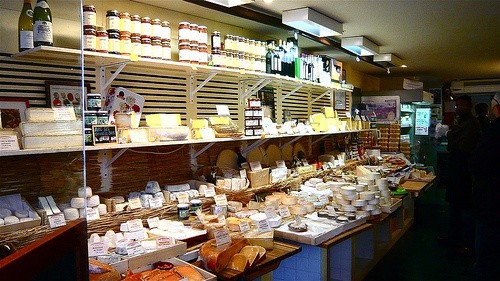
xmin=204 ymin=0 xmax=403 ymax=69
xmin=450 ymin=94 xmax=459 ymax=101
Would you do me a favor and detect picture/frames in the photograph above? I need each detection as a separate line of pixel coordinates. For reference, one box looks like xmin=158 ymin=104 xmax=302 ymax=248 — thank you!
xmin=44 ymin=80 xmax=92 ymax=114
xmin=0 ymin=98 xmax=29 ymax=128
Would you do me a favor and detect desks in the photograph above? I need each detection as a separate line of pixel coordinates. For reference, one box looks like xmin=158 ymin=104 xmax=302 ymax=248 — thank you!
xmin=433 ymin=141 xmax=448 ymax=152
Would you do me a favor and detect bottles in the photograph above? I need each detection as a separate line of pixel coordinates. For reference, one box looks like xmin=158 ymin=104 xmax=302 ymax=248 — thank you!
xmin=32 ymin=0 xmax=54 ymax=47
xmin=17 ymin=0 xmax=34 ymax=51
xmin=267 ymin=40 xmax=332 ymax=84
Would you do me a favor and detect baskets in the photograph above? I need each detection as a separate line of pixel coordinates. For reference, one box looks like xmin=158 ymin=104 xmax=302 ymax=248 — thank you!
xmin=247 ymin=170 xmax=317 ymax=193
xmin=84 ymin=189 xmax=255 ymax=239
xmin=0 ymin=224 xmax=53 ymax=254
xmin=317 ymin=158 xmax=369 ymax=177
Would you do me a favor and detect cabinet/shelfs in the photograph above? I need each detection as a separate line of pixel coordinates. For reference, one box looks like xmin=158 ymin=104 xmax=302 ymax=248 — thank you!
xmin=0 ymin=0 xmax=415 ymax=281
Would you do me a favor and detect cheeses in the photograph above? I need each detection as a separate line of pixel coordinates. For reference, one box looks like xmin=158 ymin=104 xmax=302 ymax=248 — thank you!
xmin=0 ymin=103 xmax=426 ymax=281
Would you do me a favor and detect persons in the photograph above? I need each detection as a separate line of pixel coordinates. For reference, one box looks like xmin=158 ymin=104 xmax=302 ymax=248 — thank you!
xmin=471 ymin=91 xmax=500 ymax=281
xmin=436 ymin=94 xmax=490 ymax=254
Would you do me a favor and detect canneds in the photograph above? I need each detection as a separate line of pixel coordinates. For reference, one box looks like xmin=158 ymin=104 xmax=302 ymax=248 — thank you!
xmin=177 ymin=23 xmax=266 ymax=73
xmin=81 ymin=4 xmax=172 ymax=61
xmin=83 ymin=93 xmax=109 ymax=145
